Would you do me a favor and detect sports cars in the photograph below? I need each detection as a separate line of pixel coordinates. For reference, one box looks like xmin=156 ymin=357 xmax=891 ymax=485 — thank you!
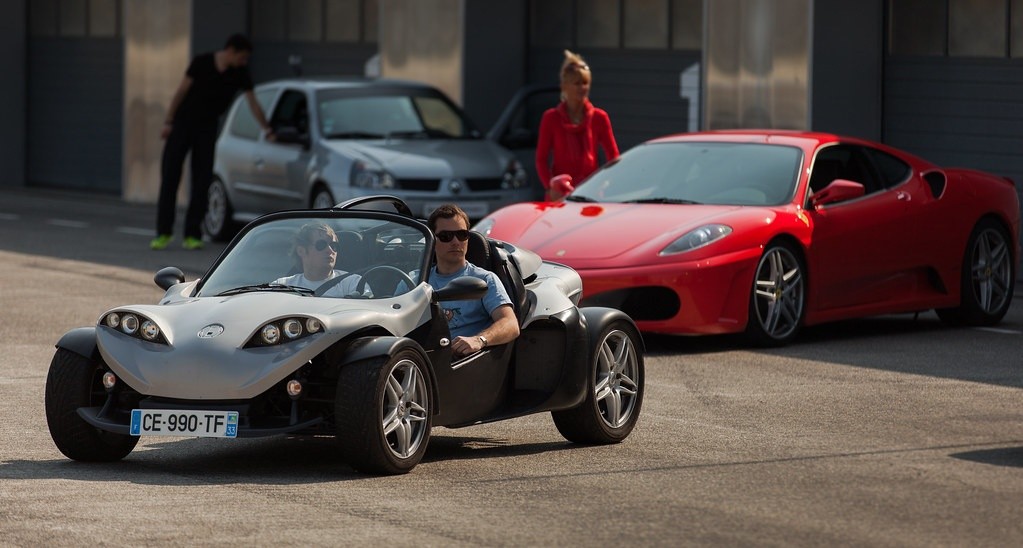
xmin=44 ymin=194 xmax=645 ymax=474
xmin=468 ymin=128 xmax=1021 ymax=349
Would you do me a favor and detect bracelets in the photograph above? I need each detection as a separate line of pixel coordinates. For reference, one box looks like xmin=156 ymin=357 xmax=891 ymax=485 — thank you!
xmin=478 ymin=335 xmax=488 ymax=349
xmin=164 ymin=119 xmax=172 ymax=125
xmin=261 ymin=127 xmax=272 ymax=137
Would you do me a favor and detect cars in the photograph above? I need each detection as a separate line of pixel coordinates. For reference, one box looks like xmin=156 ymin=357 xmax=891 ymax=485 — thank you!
xmin=204 ymin=76 xmax=535 ymax=241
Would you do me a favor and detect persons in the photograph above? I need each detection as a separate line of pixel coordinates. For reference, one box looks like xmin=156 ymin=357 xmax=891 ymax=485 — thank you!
xmin=392 ymin=203 xmax=521 ymax=360
xmin=151 ymin=32 xmax=278 ymax=251
xmin=268 ymin=221 xmax=374 ymax=299
xmin=535 ymin=50 xmax=619 ymax=202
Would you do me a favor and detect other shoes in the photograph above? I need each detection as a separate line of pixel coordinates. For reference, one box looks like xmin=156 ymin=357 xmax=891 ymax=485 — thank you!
xmin=150 ymin=234 xmax=176 ymax=250
xmin=181 ymin=238 xmax=204 ymax=251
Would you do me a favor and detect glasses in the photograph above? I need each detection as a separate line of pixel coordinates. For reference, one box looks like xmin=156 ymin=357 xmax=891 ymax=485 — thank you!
xmin=306 ymin=240 xmax=340 ymax=253
xmin=432 ymin=229 xmax=470 ymax=243
